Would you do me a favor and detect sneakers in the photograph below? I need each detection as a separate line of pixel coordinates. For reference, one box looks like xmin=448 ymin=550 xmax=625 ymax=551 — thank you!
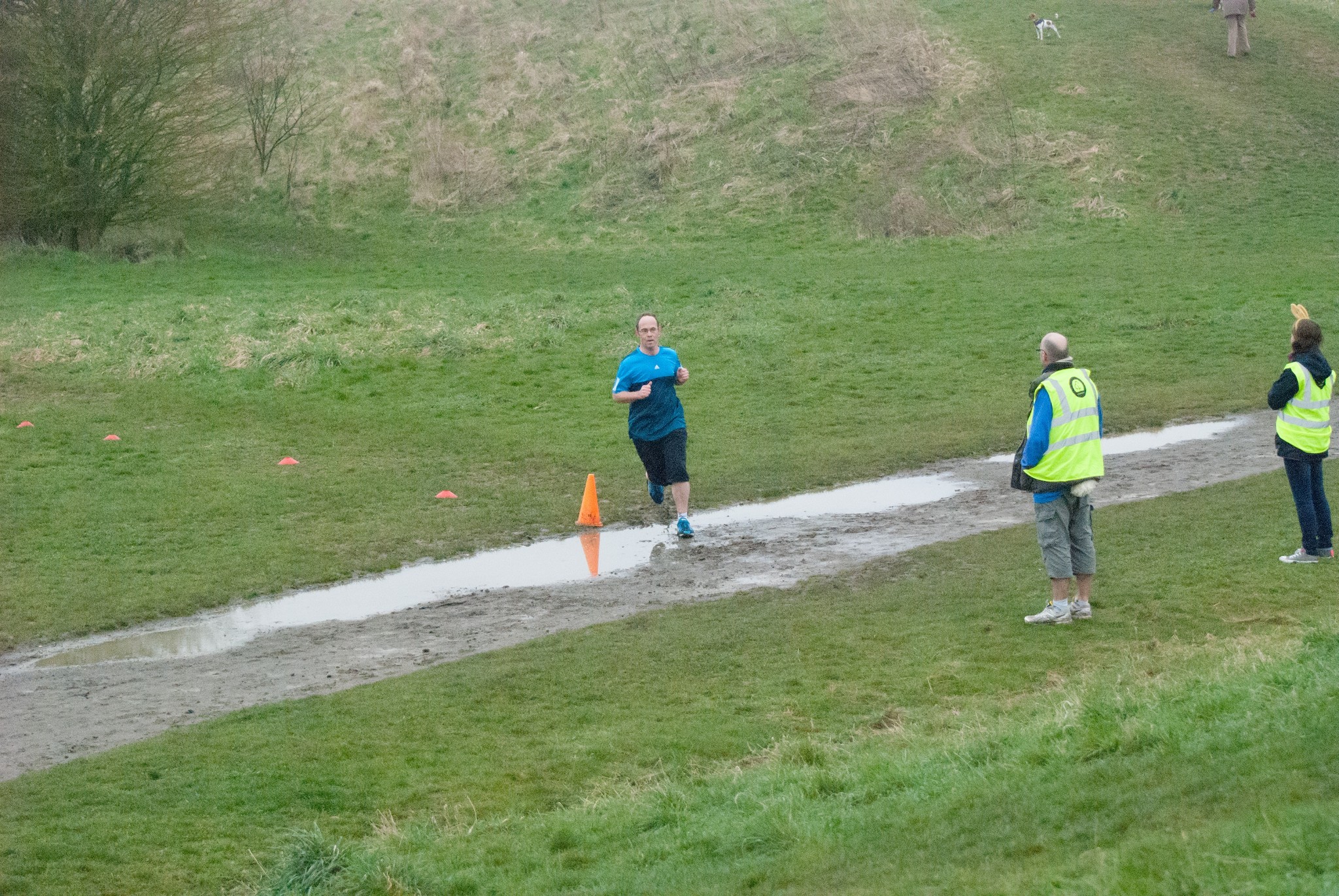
xmin=1295 ymin=547 xmax=1335 ymax=558
xmin=1024 ymin=601 xmax=1072 ymax=625
xmin=1070 ymin=597 xmax=1092 ymax=619
xmin=1279 ymin=547 xmax=1319 ymax=563
xmin=677 ymin=518 xmax=694 ymax=538
xmin=647 ymin=480 xmax=664 ymax=504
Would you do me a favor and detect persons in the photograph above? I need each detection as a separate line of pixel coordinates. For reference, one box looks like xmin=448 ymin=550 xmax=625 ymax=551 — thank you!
xmin=1210 ymin=0 xmax=1257 ymax=58
xmin=1010 ymin=332 xmax=1105 ymax=623
xmin=1267 ymin=303 xmax=1336 ymax=565
xmin=613 ymin=313 xmax=695 ymax=537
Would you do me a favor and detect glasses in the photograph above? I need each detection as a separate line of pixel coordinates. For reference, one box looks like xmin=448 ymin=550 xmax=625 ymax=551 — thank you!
xmin=1036 ymin=348 xmax=1047 ymax=356
xmin=637 ymin=328 xmax=658 ymax=334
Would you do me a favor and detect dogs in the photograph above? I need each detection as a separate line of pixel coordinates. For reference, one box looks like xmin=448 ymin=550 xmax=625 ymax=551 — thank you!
xmin=1027 ymin=12 xmax=1061 ymax=40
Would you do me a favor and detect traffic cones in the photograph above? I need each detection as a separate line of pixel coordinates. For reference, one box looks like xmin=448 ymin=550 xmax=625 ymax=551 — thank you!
xmin=574 ymin=473 xmax=604 ymax=527
xmin=579 ymin=530 xmax=601 ymax=577
